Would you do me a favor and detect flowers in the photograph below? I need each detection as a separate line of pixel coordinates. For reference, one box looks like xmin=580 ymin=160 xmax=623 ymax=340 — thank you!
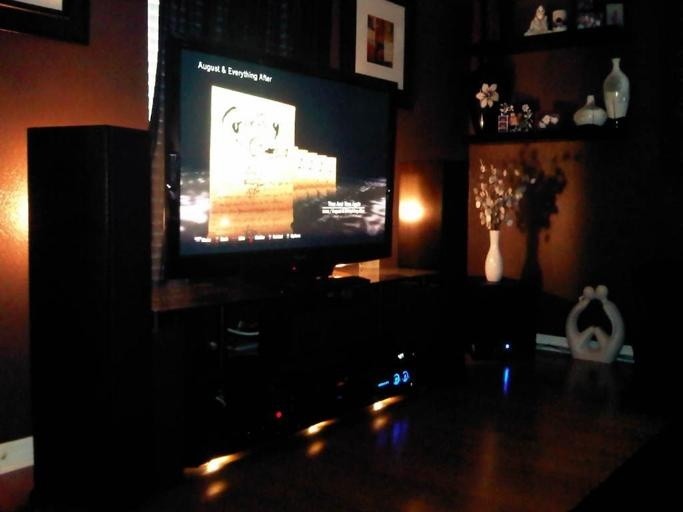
xmin=474 ymin=80 xmax=501 ymax=110
xmin=473 ymin=158 xmax=537 ymax=230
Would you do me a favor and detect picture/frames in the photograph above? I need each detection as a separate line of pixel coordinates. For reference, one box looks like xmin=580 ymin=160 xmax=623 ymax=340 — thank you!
xmin=320 ymin=0 xmax=418 ymax=100
xmin=0 ymin=0 xmax=90 ymax=46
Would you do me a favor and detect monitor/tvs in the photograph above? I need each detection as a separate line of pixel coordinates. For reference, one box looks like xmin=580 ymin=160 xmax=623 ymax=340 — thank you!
xmin=172 ymin=43 xmax=399 ymax=270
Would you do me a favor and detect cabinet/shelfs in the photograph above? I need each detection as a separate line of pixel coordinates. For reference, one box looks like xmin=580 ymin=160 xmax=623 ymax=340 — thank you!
xmin=466 ymin=0 xmax=682 ymax=145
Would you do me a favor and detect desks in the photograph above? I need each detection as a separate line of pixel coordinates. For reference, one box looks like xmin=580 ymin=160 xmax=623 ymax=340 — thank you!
xmin=152 ymin=257 xmax=439 ymax=486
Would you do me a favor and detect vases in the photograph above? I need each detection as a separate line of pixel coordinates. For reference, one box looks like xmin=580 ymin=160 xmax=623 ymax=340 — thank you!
xmin=603 ymin=56 xmax=631 ymax=121
xmin=573 ymin=95 xmax=609 ymax=127
xmin=484 ymin=229 xmax=503 ymax=282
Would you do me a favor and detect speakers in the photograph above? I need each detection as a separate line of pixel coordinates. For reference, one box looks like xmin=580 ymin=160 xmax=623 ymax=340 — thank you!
xmin=399 ymin=160 xmax=465 ymax=269
xmin=25 ymin=127 xmax=155 ymax=512
